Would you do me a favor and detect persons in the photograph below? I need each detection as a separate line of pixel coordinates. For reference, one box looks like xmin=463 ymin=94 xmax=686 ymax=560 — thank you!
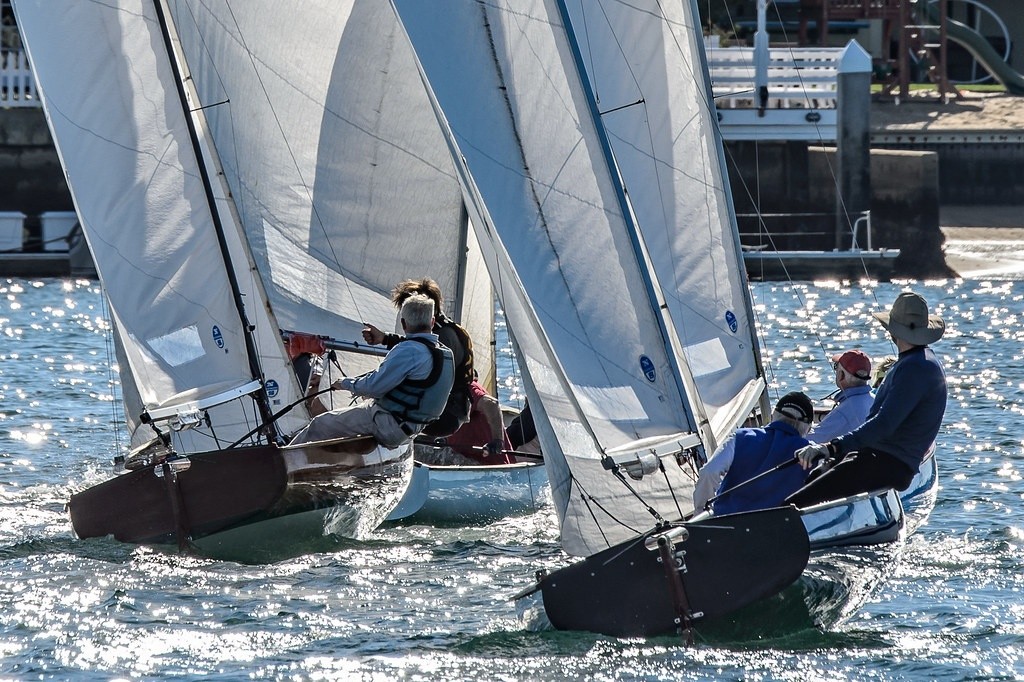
xmin=289 ymin=276 xmax=509 ymax=465
xmin=693 ymin=292 xmax=947 ymax=515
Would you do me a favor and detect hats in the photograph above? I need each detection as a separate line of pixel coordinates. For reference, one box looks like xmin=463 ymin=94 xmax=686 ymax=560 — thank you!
xmin=831 ymin=351 xmax=871 ymax=379
xmin=871 ymin=292 xmax=945 ymax=345
xmin=774 ymin=392 xmax=814 ymax=423
xmin=872 ymin=358 xmax=897 ymax=387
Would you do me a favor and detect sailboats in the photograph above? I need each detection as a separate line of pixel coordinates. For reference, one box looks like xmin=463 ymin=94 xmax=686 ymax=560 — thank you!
xmin=389 ymin=0 xmax=939 ymax=631
xmin=0 ymin=0 xmax=550 ymax=570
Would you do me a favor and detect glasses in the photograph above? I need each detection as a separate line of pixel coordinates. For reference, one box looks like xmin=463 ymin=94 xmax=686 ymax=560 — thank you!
xmin=833 ymin=362 xmax=838 ymax=369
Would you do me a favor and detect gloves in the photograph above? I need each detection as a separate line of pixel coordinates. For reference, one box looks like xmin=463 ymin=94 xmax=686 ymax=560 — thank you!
xmin=487 ymin=438 xmax=503 ymax=455
xmin=793 ymin=441 xmax=835 ymax=470
